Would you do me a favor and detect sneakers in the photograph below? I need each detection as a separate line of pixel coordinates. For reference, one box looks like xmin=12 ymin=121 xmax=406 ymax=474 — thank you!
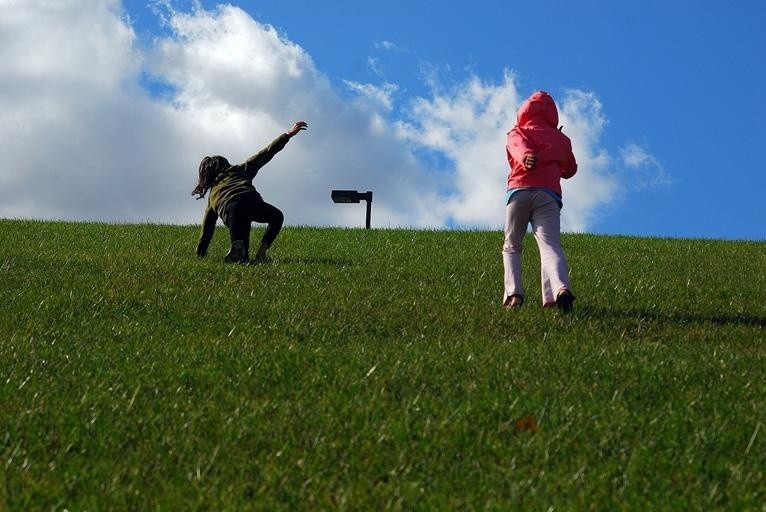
xmin=556 ymin=289 xmax=573 ymax=310
xmin=224 ymin=239 xmax=244 ymax=261
xmin=506 ymin=297 xmax=522 ymax=311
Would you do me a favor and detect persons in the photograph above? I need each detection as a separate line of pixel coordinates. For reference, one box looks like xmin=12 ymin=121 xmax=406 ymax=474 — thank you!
xmin=196 ymin=122 xmax=308 ymax=261
xmin=502 ymin=91 xmax=577 ymax=310
